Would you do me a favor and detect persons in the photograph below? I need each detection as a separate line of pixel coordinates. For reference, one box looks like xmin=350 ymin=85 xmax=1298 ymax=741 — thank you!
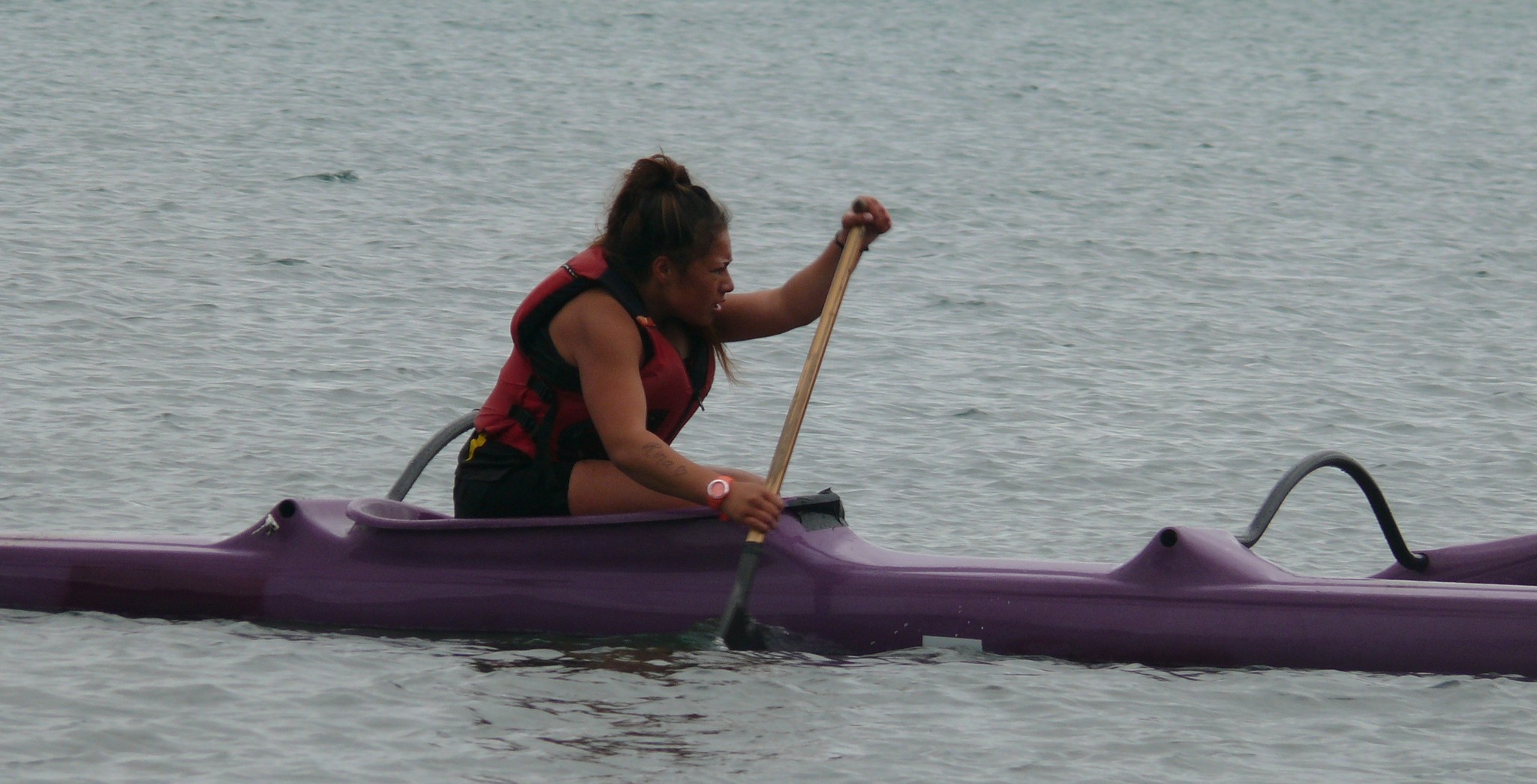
xmin=452 ymin=155 xmax=892 ymax=534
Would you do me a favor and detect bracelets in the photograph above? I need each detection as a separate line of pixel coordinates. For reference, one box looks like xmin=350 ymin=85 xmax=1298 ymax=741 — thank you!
xmin=835 ymin=229 xmax=870 ymax=252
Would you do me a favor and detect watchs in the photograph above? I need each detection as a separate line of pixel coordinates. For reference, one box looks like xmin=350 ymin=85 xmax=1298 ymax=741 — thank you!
xmin=708 ymin=474 xmax=734 ymax=522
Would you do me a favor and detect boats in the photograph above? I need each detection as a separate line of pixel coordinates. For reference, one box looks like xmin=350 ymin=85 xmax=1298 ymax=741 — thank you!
xmin=0 ymin=412 xmax=1537 ymax=677
